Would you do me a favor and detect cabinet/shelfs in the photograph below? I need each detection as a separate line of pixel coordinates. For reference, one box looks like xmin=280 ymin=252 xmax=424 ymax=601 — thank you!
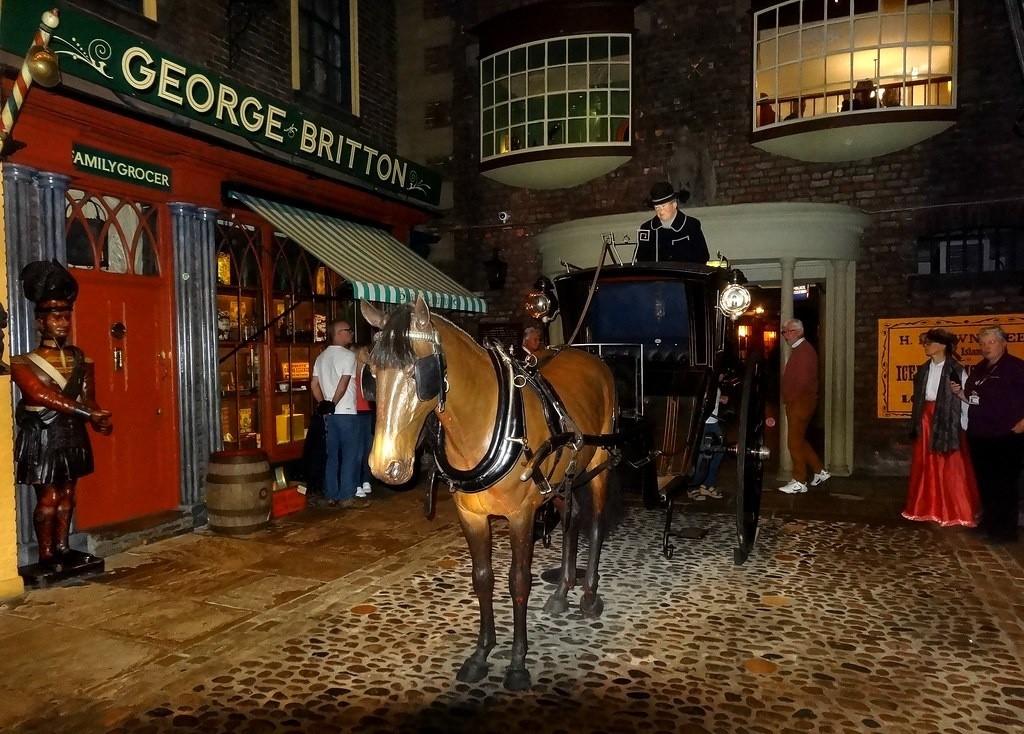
xmin=215 ymin=281 xmax=345 ymax=465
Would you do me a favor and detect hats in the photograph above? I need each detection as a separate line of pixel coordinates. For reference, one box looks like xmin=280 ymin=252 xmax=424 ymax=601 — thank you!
xmin=647 ymin=181 xmax=678 ymax=206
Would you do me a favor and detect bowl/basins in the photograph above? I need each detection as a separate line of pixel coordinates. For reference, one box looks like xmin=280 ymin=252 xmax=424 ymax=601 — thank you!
xmin=280 ymin=383 xmax=289 ymax=392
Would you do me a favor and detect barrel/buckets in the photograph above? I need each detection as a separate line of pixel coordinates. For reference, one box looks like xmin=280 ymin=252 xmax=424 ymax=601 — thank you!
xmin=207 ymin=448 xmax=273 ymax=535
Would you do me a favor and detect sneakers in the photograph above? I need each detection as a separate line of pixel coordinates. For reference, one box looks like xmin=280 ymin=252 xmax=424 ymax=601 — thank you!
xmin=810 ymin=470 xmax=830 ymax=486
xmin=778 ymin=478 xmax=807 ymax=494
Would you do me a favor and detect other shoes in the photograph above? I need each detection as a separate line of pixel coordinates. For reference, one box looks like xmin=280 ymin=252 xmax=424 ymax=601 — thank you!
xmin=355 ymin=486 xmax=366 ymax=497
xmin=984 ymin=530 xmax=1018 ymax=545
xmin=362 ymin=481 xmax=372 ymax=493
xmin=326 ymin=497 xmax=340 ymax=506
xmin=339 ymin=497 xmax=370 ymax=508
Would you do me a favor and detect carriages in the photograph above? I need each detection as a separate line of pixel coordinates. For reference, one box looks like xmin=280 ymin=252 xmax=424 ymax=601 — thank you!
xmin=356 ymin=228 xmax=773 ymax=695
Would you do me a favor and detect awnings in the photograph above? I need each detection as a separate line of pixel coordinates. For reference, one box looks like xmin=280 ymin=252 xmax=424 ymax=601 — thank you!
xmin=228 ymin=190 xmax=488 ymax=313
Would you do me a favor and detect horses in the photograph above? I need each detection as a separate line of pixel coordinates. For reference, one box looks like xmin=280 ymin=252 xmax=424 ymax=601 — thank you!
xmin=359 ymin=292 xmax=617 ymax=693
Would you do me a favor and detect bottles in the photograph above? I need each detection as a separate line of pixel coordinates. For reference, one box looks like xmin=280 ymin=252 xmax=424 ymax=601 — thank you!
xmin=241 ymin=318 xmax=259 ymax=341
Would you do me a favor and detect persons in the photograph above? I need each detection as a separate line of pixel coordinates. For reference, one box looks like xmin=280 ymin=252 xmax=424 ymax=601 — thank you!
xmin=688 ymin=375 xmax=729 ymax=501
xmin=515 ymin=327 xmax=540 ymax=361
xmin=760 ymin=93 xmax=775 ymax=126
xmin=950 ymin=325 xmax=1024 ymax=544
xmin=633 ymin=181 xmax=710 ymax=265
xmin=784 ymin=100 xmax=806 ymax=120
xmin=778 ymin=319 xmax=830 ymax=494
xmin=841 ymin=80 xmax=901 ymax=111
xmin=6 ymin=261 xmax=113 ymax=571
xmin=310 ymin=319 xmax=376 ymax=509
xmin=902 ymin=329 xmax=980 ymax=527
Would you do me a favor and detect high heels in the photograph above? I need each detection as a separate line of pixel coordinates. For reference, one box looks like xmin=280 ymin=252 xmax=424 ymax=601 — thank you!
xmin=700 ymin=484 xmax=723 ymax=498
xmin=687 ymin=490 xmax=706 ymax=500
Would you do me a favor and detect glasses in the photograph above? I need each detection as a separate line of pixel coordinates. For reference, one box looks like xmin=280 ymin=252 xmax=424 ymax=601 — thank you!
xmin=781 ymin=328 xmax=798 ymax=337
xmin=339 ymin=327 xmax=353 ymax=336
xmin=922 ymin=340 xmax=935 ymax=347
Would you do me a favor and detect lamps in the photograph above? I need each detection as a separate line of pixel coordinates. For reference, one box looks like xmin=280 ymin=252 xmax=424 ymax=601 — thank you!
xmin=505 ymin=134 xmax=509 ymax=150
xmin=483 ymin=247 xmax=507 ymax=290
xmin=26 ymin=45 xmax=60 ymax=86
